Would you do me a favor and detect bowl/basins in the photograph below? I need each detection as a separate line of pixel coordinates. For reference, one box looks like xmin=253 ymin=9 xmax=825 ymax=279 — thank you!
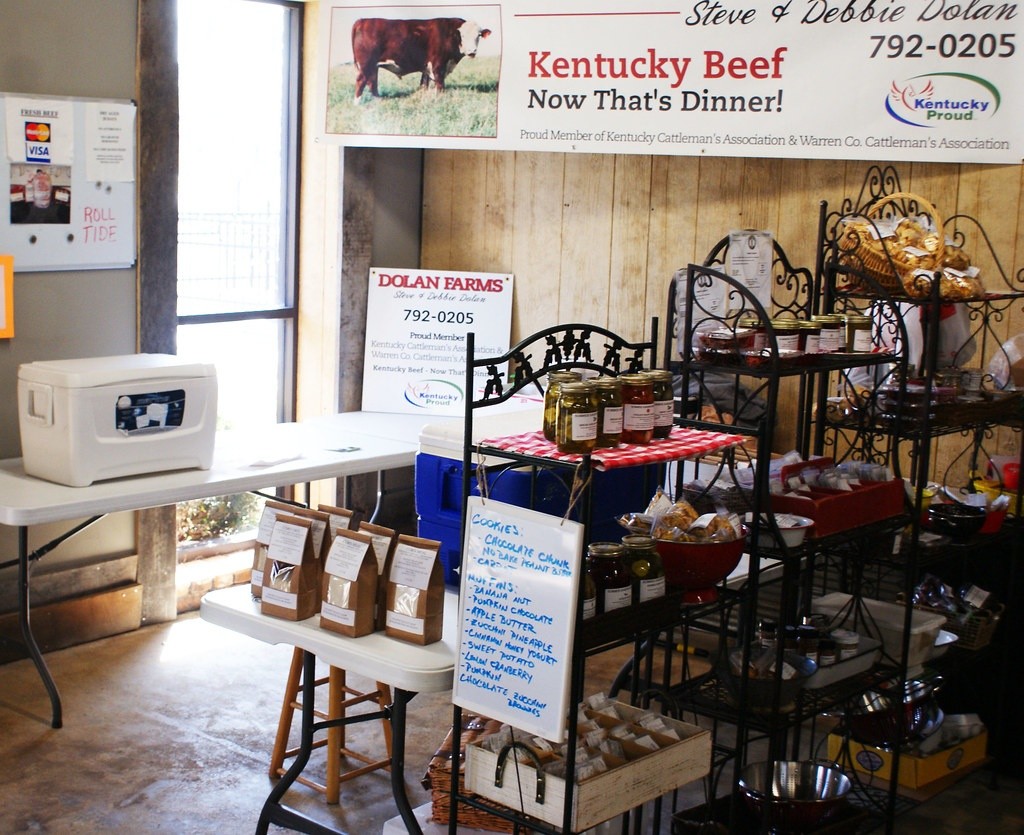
xmin=739 ymin=513 xmax=815 ymax=548
xmin=908 ymin=707 xmax=943 ymax=752
xmin=737 ymin=757 xmax=853 ymax=823
xmin=710 ymin=649 xmax=817 ymax=707
xmin=927 ymin=630 xmax=959 ymax=660
xmin=981 ymin=505 xmax=1009 ymax=534
xmin=926 ymin=503 xmax=988 ymax=535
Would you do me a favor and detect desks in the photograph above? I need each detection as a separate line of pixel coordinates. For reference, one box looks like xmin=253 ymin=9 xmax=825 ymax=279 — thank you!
xmin=199 ymin=554 xmax=457 ymax=835
xmin=0 ymin=389 xmax=546 ymax=728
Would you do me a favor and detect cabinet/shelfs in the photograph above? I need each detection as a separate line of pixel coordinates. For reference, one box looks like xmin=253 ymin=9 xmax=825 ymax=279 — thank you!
xmin=453 ymin=166 xmax=1024 ymax=835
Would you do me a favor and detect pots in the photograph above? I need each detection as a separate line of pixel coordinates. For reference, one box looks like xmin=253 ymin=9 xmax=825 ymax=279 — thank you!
xmin=821 ymin=676 xmax=943 ymax=745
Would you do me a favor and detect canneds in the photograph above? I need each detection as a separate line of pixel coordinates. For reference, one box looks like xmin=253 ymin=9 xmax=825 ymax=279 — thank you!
xmin=579 ymin=535 xmax=671 ymax=622
xmin=739 ymin=316 xmax=874 ymax=354
xmin=759 ymin=620 xmax=859 ymax=666
xmin=542 ymin=369 xmax=673 ymax=454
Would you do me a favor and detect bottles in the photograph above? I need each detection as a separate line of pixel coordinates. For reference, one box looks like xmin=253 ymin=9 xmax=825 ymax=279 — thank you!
xmin=542 ymin=368 xmax=675 ymax=455
xmin=737 ymin=314 xmax=874 ymax=354
xmin=757 ymin=613 xmax=860 ymax=667
xmin=580 ymin=533 xmax=666 ymax=620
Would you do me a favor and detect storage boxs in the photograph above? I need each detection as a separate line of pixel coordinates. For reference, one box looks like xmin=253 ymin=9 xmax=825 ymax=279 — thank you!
xmin=680 ymin=457 xmax=905 ymax=538
xmin=811 ymin=592 xmax=948 ymax=666
xmin=463 ymin=698 xmax=714 ymax=835
xmin=744 ymin=637 xmax=884 ymax=690
xmin=829 ymin=728 xmax=989 ymax=801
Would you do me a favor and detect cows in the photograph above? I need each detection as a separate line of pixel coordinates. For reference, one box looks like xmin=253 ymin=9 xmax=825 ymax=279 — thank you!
xmin=352 ymin=15 xmax=492 ymax=104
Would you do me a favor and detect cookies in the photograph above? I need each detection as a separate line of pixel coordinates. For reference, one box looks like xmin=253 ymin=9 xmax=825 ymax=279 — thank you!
xmin=849 ymin=217 xmax=982 ymax=300
